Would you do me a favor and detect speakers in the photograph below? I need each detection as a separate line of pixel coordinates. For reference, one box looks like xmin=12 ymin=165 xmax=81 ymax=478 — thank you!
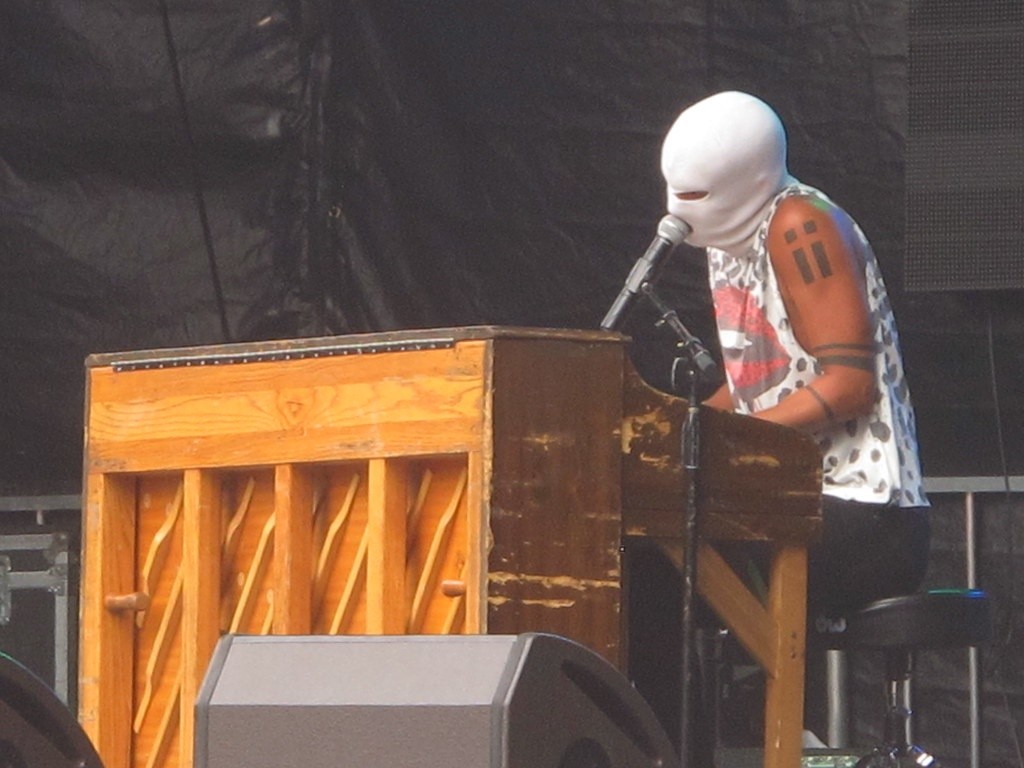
xmin=192 ymin=633 xmax=680 ymax=768
xmin=0 ymin=655 xmax=103 ymax=768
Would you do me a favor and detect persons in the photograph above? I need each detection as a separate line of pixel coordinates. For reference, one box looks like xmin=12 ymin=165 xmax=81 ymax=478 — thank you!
xmin=629 ymin=91 xmax=930 ymax=754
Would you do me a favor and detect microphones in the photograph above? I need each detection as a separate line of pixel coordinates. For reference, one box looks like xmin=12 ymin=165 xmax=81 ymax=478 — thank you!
xmin=599 ymin=215 xmax=691 ymax=332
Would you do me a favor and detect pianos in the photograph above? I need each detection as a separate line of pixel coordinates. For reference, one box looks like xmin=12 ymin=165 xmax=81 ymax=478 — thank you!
xmin=77 ymin=324 xmax=824 ymax=768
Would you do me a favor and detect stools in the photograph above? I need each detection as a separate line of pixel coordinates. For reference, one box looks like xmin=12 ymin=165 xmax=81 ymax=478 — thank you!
xmin=809 ymin=588 xmax=989 ymax=768
